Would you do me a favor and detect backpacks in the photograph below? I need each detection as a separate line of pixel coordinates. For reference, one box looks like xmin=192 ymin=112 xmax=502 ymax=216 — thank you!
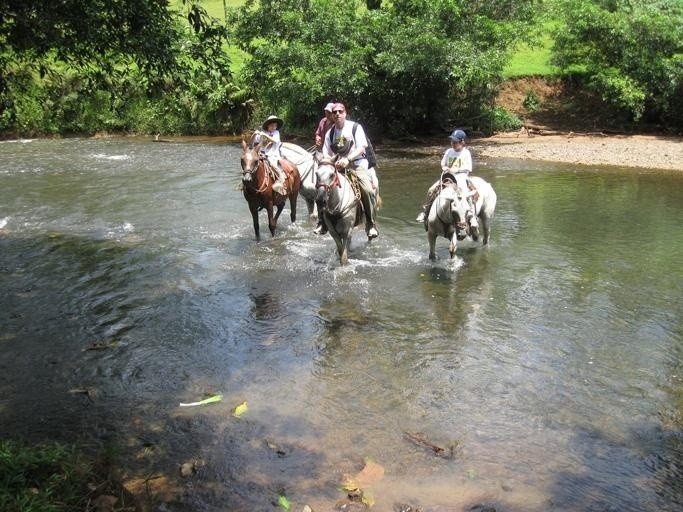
xmin=364 ymin=135 xmax=377 ymax=169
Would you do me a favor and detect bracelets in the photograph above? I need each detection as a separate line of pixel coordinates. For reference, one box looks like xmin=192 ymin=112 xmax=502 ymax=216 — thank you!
xmin=347 ymin=157 xmax=352 ymax=163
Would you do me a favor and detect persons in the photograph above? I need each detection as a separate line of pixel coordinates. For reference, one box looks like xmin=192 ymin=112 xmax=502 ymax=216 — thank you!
xmin=252 ymin=115 xmax=288 ymax=196
xmin=313 ymin=103 xmax=378 ymax=237
xmin=309 ymin=103 xmax=335 ymax=166
xmin=416 ymin=130 xmax=480 ymax=242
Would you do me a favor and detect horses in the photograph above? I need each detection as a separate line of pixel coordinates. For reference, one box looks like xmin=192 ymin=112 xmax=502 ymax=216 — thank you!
xmin=278 ymin=141 xmax=317 ymax=216
xmin=240 ymin=140 xmax=301 ymax=241
xmin=427 ymin=176 xmax=497 ymax=261
xmin=314 ymin=149 xmax=383 ymax=266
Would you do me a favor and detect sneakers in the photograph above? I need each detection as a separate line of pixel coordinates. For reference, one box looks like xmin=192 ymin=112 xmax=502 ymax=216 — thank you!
xmin=366 ymin=227 xmax=379 ymax=239
xmin=469 ymin=216 xmax=479 ymax=229
xmin=415 ymin=212 xmax=427 ymax=223
xmin=313 ymin=221 xmax=328 ymax=235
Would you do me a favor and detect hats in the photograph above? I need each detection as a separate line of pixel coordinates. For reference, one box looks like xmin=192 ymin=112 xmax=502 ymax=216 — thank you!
xmin=323 ymin=102 xmax=346 ymax=112
xmin=261 ymin=115 xmax=285 ymax=131
xmin=447 ymin=129 xmax=467 ymax=143
